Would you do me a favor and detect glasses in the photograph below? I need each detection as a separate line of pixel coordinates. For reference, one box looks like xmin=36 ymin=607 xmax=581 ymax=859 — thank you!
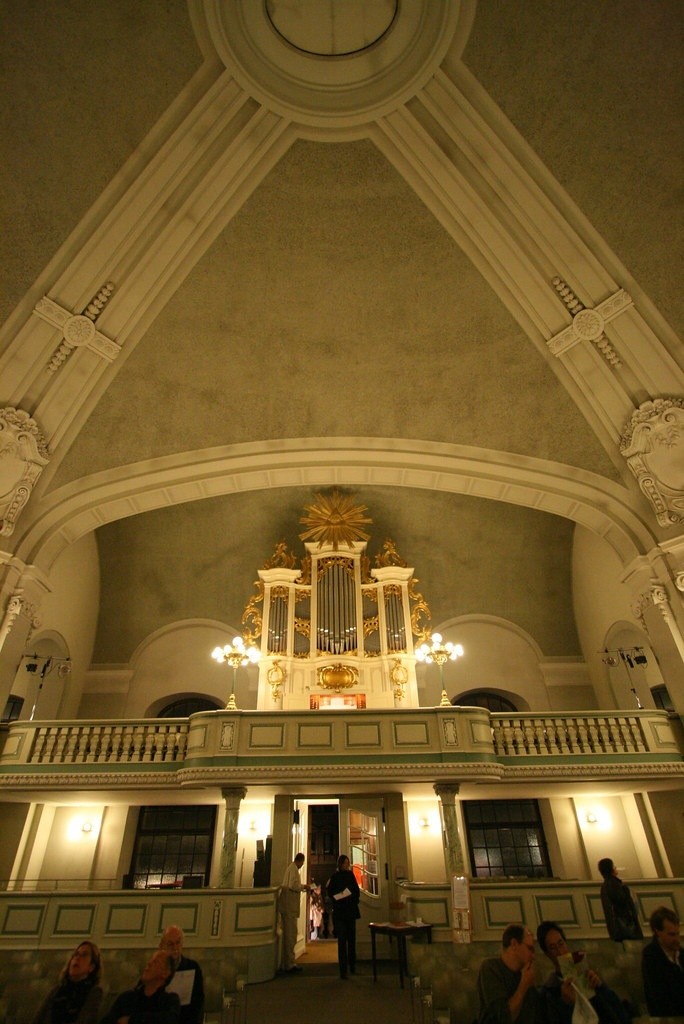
xmin=521 ymin=942 xmax=536 ymax=953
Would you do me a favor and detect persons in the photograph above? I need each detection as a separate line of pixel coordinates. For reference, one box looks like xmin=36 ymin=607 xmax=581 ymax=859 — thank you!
xmin=329 ymin=853 xmax=362 ymax=981
xmin=598 ymin=856 xmax=643 ymax=940
xmin=279 ymin=854 xmax=313 ymax=971
xmin=478 ymin=924 xmax=543 ymax=1024
xmin=30 ymin=940 xmax=103 ymax=1022
xmin=539 ymin=920 xmax=631 ymax=1024
xmin=135 ymin=922 xmax=205 ymax=1024
xmin=107 ymin=948 xmax=190 ymax=1023
xmin=641 ymin=904 xmax=684 ymax=1017
xmin=311 ymin=882 xmax=327 ymax=940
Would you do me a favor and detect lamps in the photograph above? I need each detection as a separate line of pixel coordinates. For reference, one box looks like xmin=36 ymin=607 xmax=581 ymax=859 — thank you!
xmin=210 ymin=636 xmax=261 ymax=710
xmin=414 ymin=632 xmax=464 ymax=707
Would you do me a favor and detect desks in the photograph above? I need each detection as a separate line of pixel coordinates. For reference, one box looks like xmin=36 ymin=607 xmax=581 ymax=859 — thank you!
xmin=368 ymin=920 xmax=433 ymax=990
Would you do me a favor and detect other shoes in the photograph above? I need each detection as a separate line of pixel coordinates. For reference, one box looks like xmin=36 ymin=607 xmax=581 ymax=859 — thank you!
xmin=284 ymin=966 xmax=303 ymax=974
